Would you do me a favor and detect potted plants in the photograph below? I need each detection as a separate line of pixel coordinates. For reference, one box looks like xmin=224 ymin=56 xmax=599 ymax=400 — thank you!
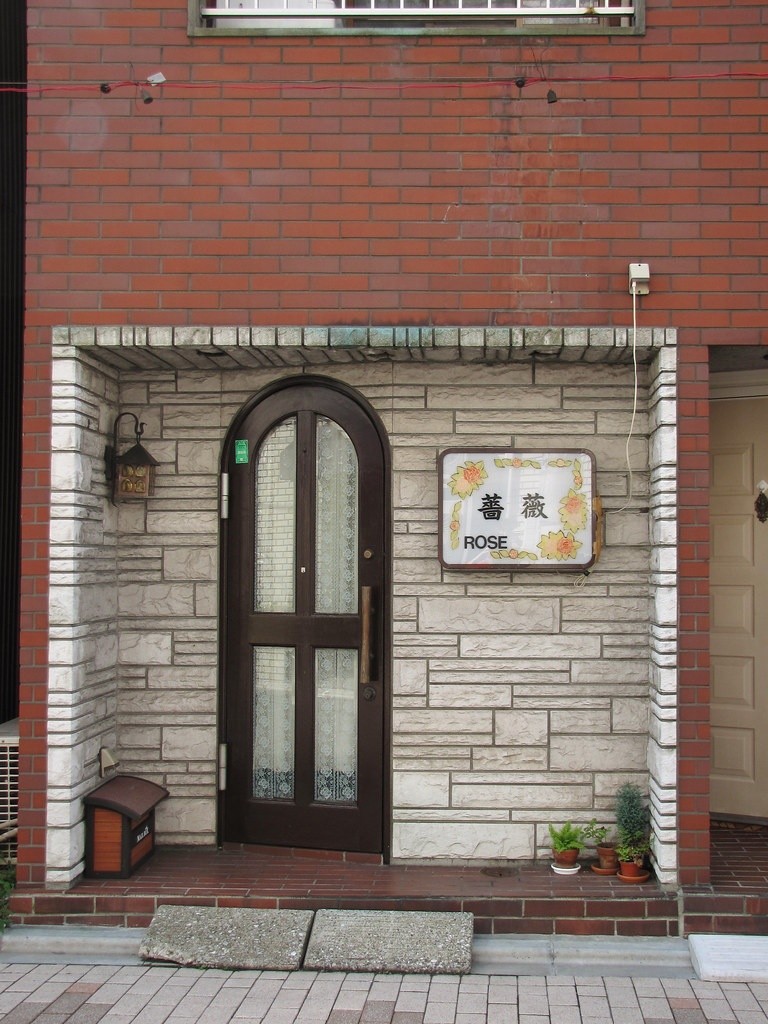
xmin=581 ymin=818 xmax=617 ymax=868
xmin=613 ymin=784 xmax=649 ymax=874
xmin=549 ymin=817 xmax=588 ymax=866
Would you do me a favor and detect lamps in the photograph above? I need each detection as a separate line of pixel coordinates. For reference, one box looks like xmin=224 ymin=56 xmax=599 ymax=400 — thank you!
xmin=106 ymin=411 xmax=161 ymax=504
